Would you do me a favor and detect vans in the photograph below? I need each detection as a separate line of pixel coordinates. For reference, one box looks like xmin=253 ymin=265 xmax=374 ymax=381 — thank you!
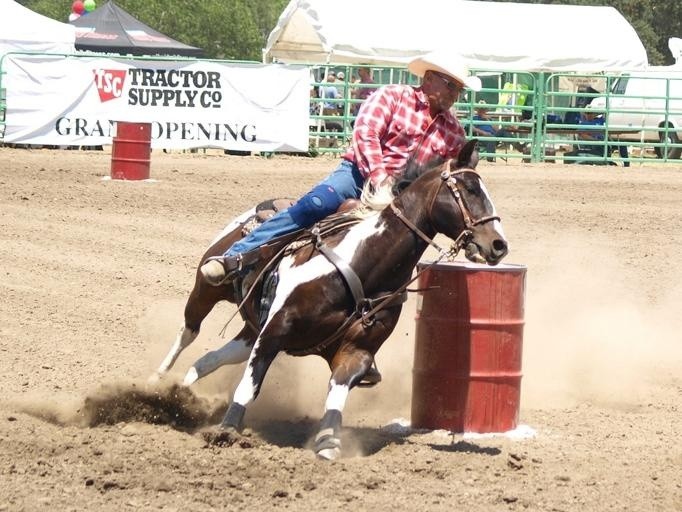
xmin=585 ymin=71 xmax=682 ymax=158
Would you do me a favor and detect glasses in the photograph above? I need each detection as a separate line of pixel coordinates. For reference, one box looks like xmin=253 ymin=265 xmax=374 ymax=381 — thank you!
xmin=432 ymin=72 xmax=466 ymax=93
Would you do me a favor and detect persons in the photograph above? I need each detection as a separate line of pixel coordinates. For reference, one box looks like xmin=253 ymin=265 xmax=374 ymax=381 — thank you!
xmin=577 ymin=106 xmax=630 ymax=167
xmin=465 ymin=99 xmax=529 ymax=162
xmin=309 ymin=62 xmax=377 ymax=146
xmin=201 ymin=54 xmax=482 ymax=384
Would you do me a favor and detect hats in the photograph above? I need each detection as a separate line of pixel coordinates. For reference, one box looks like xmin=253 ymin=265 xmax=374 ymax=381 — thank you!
xmin=473 ymin=100 xmax=491 ymax=111
xmin=408 ymin=51 xmax=482 ymax=92
xmin=337 ymin=72 xmax=345 ymax=78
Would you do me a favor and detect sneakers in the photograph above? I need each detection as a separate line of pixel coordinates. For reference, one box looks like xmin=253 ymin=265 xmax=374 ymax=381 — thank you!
xmin=363 ymin=367 xmax=381 ymax=385
xmin=201 ymin=259 xmax=232 ymax=287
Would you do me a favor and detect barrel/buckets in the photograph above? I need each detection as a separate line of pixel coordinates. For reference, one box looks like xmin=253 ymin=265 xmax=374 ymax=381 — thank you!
xmin=411 ymin=262 xmax=527 ymax=434
xmin=111 ymin=121 xmax=152 ymax=180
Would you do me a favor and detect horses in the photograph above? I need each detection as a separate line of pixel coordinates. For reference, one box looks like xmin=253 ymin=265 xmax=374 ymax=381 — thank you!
xmin=146 ymin=137 xmax=509 ymax=463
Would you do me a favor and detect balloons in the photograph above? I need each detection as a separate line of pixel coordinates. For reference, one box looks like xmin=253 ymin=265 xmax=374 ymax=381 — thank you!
xmin=68 ymin=0 xmax=96 ymax=22
